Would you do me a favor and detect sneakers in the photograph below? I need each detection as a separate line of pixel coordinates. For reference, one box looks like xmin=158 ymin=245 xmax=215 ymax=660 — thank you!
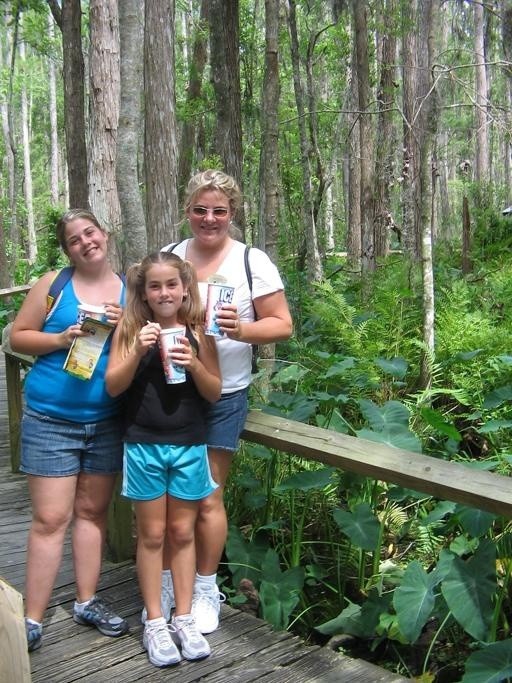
xmin=23 ymin=583 xmax=225 ymax=669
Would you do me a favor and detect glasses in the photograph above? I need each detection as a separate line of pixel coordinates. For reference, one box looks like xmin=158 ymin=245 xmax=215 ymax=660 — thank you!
xmin=192 ymin=206 xmax=227 ymax=219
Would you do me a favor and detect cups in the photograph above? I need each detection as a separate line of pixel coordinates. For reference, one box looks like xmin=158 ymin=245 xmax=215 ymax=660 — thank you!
xmin=155 ymin=326 xmax=189 ymax=386
xmin=75 ymin=303 xmax=107 ymax=329
xmin=203 ymin=283 xmax=236 ymax=338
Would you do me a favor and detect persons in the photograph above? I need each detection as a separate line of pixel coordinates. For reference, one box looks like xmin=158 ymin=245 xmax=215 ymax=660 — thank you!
xmin=139 ymin=169 xmax=294 ymax=636
xmin=8 ymin=207 xmax=130 ymax=653
xmin=104 ymin=251 xmax=224 ymax=667
